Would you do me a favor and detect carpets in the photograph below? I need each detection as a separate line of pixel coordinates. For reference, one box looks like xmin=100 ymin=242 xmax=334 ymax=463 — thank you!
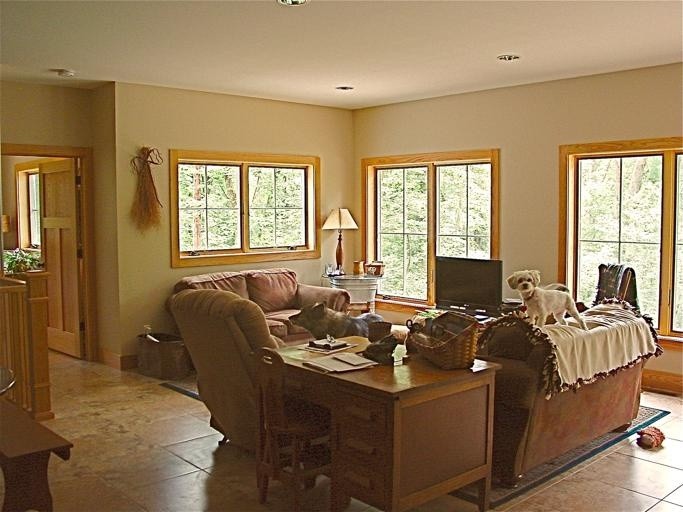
xmin=159 ymin=326 xmax=671 ymax=509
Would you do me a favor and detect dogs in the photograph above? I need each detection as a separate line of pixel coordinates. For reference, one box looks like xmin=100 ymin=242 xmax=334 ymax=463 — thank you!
xmin=506 ymin=270 xmax=589 ymax=331
xmin=289 ymin=299 xmax=387 ymax=341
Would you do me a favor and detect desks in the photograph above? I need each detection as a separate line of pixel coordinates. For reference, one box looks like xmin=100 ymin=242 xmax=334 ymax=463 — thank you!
xmin=0 ymin=395 xmax=74 ymax=511
xmin=251 ymin=348 xmax=494 ymax=512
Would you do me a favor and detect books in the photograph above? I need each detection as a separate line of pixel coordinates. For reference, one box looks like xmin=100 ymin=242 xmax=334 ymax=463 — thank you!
xmin=302 ymin=353 xmax=379 ymax=374
xmin=308 ymin=338 xmax=347 ymax=350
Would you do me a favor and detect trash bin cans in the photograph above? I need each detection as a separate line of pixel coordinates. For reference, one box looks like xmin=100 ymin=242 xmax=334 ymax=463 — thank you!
xmin=137 ymin=332 xmax=192 ymax=380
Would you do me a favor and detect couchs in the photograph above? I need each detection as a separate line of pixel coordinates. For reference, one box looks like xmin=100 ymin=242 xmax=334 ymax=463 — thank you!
xmin=478 ymin=302 xmax=644 ymax=489
xmin=165 ymin=267 xmax=350 ymax=446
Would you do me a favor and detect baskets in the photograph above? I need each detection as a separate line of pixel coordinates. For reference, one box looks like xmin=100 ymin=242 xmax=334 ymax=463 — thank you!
xmin=368 ymin=322 xmax=392 ymax=342
xmin=410 ymin=310 xmax=478 ymax=371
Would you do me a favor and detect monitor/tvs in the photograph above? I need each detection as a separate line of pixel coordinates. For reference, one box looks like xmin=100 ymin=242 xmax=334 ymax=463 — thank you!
xmin=435 ymin=256 xmax=502 ymax=316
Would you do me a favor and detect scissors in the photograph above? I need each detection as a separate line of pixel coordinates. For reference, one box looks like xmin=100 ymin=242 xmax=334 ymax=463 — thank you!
xmin=406 ymin=319 xmax=421 ymax=334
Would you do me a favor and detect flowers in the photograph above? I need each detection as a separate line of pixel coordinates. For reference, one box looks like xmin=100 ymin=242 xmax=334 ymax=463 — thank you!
xmin=2 ymin=248 xmax=42 ymax=272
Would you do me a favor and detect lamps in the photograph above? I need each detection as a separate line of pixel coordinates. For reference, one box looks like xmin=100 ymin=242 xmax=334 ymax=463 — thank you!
xmin=321 ymin=207 xmax=358 ymax=272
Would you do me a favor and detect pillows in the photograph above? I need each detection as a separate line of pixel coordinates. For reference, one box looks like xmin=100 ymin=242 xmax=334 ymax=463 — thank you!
xmin=291 ymin=299 xmax=388 ymax=338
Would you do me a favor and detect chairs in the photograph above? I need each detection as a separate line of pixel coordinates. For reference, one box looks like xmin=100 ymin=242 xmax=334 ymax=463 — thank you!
xmin=574 ymin=265 xmax=637 ymax=317
xmin=253 ymin=347 xmax=330 ymax=511
xmin=167 ymin=288 xmax=289 ymax=448
